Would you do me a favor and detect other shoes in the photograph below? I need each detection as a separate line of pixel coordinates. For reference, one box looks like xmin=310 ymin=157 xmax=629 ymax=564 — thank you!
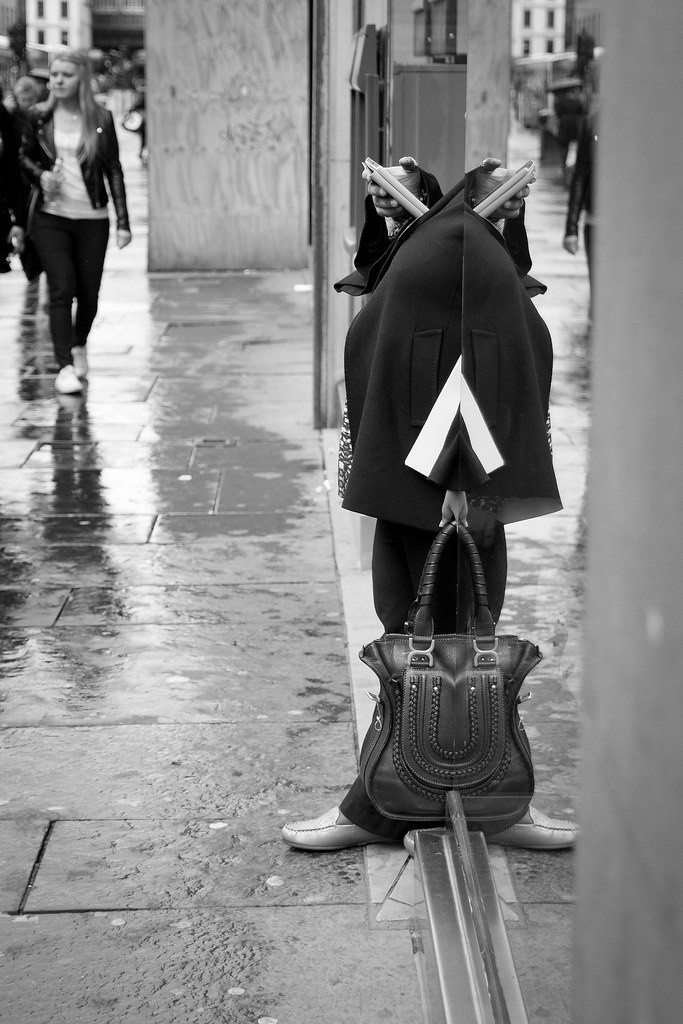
xmin=282 ymin=806 xmax=399 ymax=850
xmin=404 ymin=828 xmax=445 ymax=856
xmin=71 ymin=345 xmax=89 ymax=379
xmin=53 ymin=363 xmax=83 ymax=395
xmin=486 ymin=807 xmax=579 ymax=849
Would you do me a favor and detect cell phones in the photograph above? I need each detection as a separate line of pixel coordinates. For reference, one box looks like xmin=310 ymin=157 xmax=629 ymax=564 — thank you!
xmin=473 ymin=161 xmax=535 ymax=214
xmin=366 ymin=157 xmax=429 ymax=214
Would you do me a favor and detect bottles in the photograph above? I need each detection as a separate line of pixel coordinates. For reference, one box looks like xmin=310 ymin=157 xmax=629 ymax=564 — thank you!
xmin=43 ymin=158 xmax=63 ymax=205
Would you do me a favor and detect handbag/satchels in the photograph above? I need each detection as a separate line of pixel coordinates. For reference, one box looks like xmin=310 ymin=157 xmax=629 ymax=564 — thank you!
xmin=358 ymin=524 xmax=456 ymax=822
xmin=456 ymin=521 xmax=544 ymax=832
xmin=19 ymin=240 xmax=44 ymax=282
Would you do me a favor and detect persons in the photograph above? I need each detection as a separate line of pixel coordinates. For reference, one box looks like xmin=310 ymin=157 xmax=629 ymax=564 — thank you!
xmin=0 ymin=74 xmax=51 ymax=283
xmin=18 ymin=51 xmax=133 ymax=394
xmin=281 ymin=155 xmax=465 ymax=852
xmin=561 ymin=111 xmax=596 ymax=322
xmin=459 ymin=158 xmax=580 ymax=852
